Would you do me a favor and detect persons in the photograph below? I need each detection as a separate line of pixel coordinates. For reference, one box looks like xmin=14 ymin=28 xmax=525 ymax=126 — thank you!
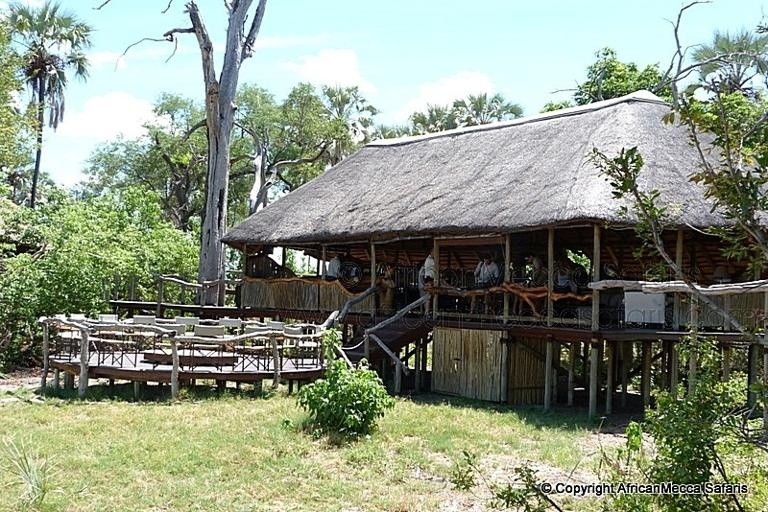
xmin=326 ymin=247 xmax=359 ymax=279
xmin=418 ymin=244 xmax=434 ymax=314
xmin=464 ymin=252 xmax=500 ymax=322
xmin=526 ymin=251 xmax=544 ymax=279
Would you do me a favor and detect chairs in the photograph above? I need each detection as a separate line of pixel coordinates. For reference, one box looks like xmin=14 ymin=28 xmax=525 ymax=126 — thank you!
xmin=56 ymin=310 xmax=338 ymax=370
